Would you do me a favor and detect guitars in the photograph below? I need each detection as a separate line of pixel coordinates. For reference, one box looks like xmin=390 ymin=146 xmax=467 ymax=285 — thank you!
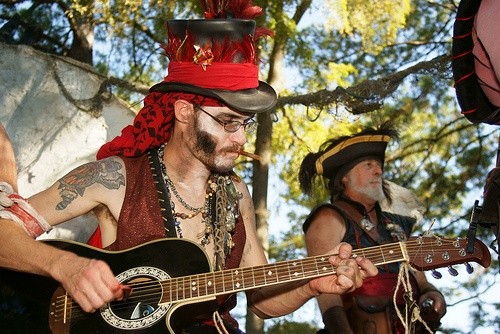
xmin=0 ymin=199 xmax=493 ymax=334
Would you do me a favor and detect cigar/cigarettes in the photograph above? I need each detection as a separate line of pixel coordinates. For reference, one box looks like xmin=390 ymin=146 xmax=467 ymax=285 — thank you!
xmin=238 ymin=151 xmax=262 ymax=160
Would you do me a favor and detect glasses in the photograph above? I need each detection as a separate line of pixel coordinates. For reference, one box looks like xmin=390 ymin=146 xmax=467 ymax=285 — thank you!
xmin=192 ymin=102 xmax=260 ymax=133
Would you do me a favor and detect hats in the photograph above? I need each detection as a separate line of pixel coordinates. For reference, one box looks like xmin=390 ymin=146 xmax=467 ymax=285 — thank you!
xmin=298 ymin=120 xmax=401 ymax=196
xmin=149 ymin=18 xmax=279 ymax=113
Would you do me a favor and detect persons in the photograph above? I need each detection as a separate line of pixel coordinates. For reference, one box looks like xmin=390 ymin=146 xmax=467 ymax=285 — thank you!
xmin=0 ymin=0 xmax=378 ymax=334
xmin=299 ymin=121 xmax=446 ymax=334
xmin=0 ymin=121 xmax=19 ymax=196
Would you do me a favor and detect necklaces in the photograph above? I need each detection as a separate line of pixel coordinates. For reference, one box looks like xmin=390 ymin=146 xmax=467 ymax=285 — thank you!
xmin=157 ymin=142 xmax=218 ymax=248
